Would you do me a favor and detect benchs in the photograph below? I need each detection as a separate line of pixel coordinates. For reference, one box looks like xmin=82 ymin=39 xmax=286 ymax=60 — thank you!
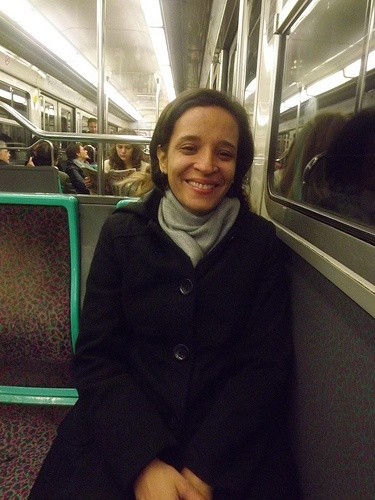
xmin=0 ymin=191 xmax=138 ymax=499
xmin=1 ymin=165 xmax=61 ymax=193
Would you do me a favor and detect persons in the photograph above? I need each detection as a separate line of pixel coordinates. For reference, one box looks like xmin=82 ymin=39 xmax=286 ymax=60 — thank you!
xmin=29 ymin=89 xmax=304 ymax=494
xmin=1 ymin=119 xmax=154 ymax=196
